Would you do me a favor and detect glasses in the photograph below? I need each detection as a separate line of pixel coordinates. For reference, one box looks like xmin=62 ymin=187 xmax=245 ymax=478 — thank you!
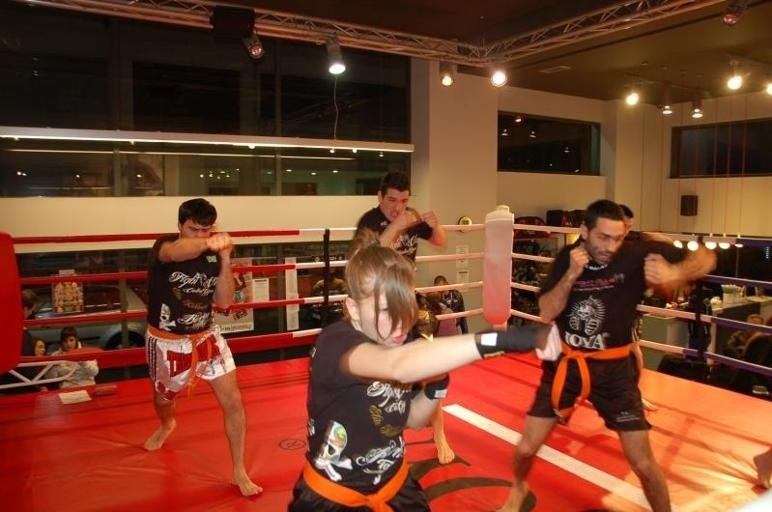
xmin=324 ymin=33 xmax=346 ymax=75
xmin=440 ymin=59 xmax=454 ymax=87
xmin=242 ymin=30 xmax=264 ymax=60
xmin=673 ymin=232 xmax=744 ymax=252
xmin=726 ymin=51 xmax=772 ymax=97
xmin=723 ymin=0 xmax=754 ymax=26
xmin=488 ymin=64 xmax=509 ymax=87
xmin=621 ymin=68 xmax=712 ymax=119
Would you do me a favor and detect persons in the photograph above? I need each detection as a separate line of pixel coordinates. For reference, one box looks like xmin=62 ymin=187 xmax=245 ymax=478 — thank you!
xmin=495 ymin=196 xmax=719 ymax=512
xmin=44 ymin=325 xmax=102 ymax=388
xmin=617 ymin=205 xmax=661 ymax=413
xmin=310 ymin=268 xmax=348 ymax=325
xmin=281 ymin=225 xmax=564 ymax=512
xmin=426 ymin=292 xmax=456 ymax=337
xmin=433 ymin=275 xmax=469 ymax=333
xmin=26 ymin=334 xmax=53 ymax=393
xmin=22 ymin=288 xmax=36 ymax=353
xmin=670 ymin=280 xmax=771 ymax=363
xmin=115 ymin=146 xmax=167 ymax=194
xmin=749 ymin=442 xmax=772 ymax=489
xmin=137 ymin=194 xmax=264 ymax=498
xmin=351 ymin=170 xmax=458 ymax=467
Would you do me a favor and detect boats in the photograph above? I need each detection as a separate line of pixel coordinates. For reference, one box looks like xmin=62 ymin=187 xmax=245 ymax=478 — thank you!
xmin=214 ymin=6 xmax=256 ymax=43
xmin=679 ymin=195 xmax=697 ymax=216
xmin=547 ymin=210 xmax=563 ymax=233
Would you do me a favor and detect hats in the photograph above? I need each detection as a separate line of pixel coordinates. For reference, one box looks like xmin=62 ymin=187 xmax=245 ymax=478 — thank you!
xmin=19 ymin=281 xmax=147 ymax=351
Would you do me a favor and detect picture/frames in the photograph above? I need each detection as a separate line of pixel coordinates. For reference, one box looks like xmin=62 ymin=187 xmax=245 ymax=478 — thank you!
xmin=641 ymin=294 xmax=772 ymax=370
